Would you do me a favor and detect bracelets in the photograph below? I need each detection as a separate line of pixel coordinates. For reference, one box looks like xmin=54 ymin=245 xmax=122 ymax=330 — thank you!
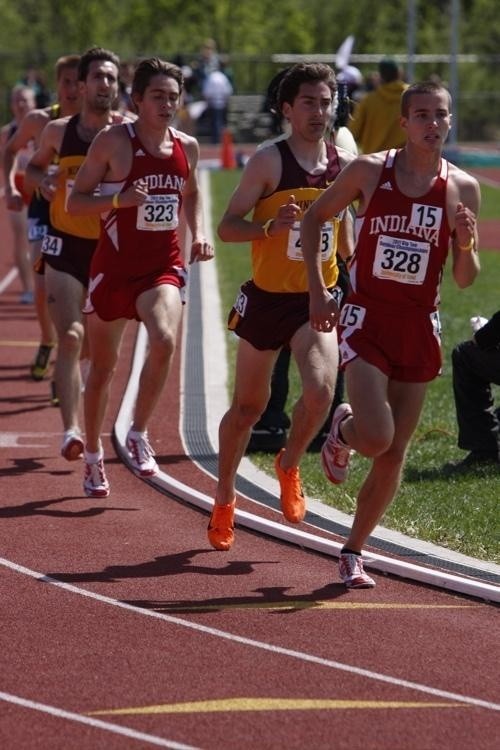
xmin=458 ymin=238 xmax=475 ymax=252
xmin=261 ymin=218 xmax=275 ymax=238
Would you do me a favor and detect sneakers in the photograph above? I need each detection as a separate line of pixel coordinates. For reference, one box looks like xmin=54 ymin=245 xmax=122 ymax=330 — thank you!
xmin=208 ymin=497 xmax=237 ymax=551
xmin=338 ymin=553 xmax=377 ymax=589
xmin=125 ymin=434 xmax=158 ymax=479
xmin=32 ymin=342 xmax=54 ymax=378
xmin=61 ymin=428 xmax=84 ymax=461
xmin=274 ymin=449 xmax=308 ymax=523
xmin=83 ymin=455 xmax=109 ymax=498
xmin=321 ymin=403 xmax=353 ymax=485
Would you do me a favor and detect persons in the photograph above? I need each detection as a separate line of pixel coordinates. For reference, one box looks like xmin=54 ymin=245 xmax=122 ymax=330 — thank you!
xmin=4 ymin=38 xmax=413 ymax=504
xmin=209 ymin=59 xmax=359 ymax=553
xmin=300 ymin=81 xmax=485 ymax=594
